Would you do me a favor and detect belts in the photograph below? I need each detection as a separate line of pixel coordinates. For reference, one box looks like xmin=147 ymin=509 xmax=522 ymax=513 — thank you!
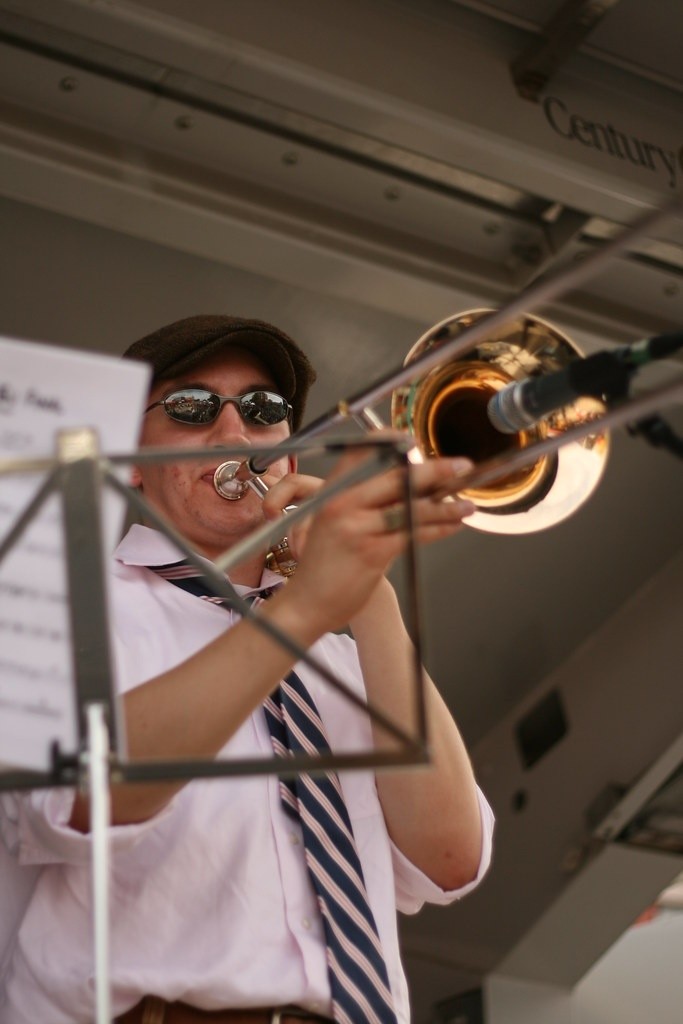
xmin=143 ymin=1003 xmax=339 ymax=1024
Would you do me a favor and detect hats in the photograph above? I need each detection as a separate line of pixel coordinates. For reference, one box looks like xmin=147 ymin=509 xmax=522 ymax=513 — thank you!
xmin=121 ymin=314 xmax=317 ymax=433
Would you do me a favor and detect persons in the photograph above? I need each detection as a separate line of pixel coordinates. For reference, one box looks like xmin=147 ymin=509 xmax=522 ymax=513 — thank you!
xmin=0 ymin=315 xmax=496 ymax=1024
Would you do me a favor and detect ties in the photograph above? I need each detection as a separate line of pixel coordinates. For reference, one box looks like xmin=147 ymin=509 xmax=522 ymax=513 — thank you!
xmin=148 ymin=558 xmax=397 ymax=1023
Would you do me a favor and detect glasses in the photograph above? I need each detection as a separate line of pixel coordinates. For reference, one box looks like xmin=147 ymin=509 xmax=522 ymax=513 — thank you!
xmin=144 ymin=388 xmax=293 ymax=426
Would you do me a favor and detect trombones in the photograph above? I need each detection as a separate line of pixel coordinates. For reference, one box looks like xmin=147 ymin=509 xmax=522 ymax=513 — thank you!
xmin=211 ymin=192 xmax=680 ymax=540
